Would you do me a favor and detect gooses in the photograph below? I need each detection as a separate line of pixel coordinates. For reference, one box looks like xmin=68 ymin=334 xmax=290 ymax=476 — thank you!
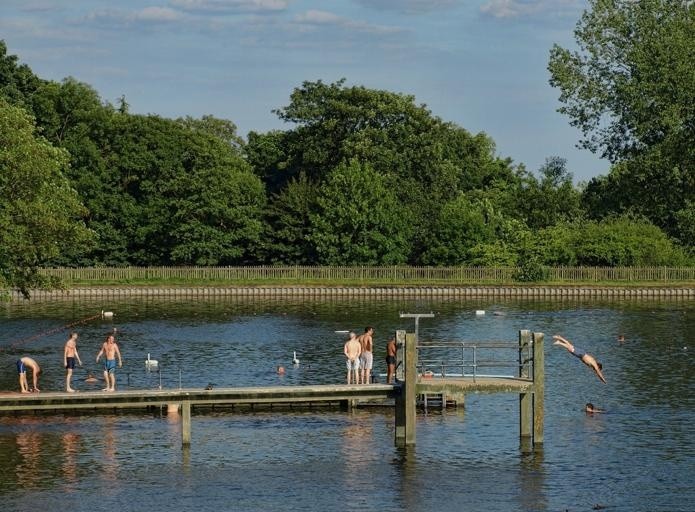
xmin=101 ymin=309 xmax=114 ymax=316
xmin=291 ymin=350 xmax=300 ymax=364
xmin=145 ymin=351 xmax=159 ymax=364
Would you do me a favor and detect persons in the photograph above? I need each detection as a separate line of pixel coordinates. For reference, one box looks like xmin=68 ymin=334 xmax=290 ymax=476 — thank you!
xmin=343 ymin=331 xmax=361 ymax=386
xmin=14 ymin=419 xmax=121 ymax=494
xmin=96 ymin=334 xmax=123 ymax=392
xmin=17 ymin=356 xmax=42 ymax=394
xmin=618 ymin=335 xmax=626 ymax=340
xmin=585 ymin=404 xmax=605 ymax=413
xmin=357 ymin=326 xmax=373 ymax=384
xmin=85 ymin=373 xmax=97 ymax=383
xmin=277 ymin=365 xmax=285 ymax=373
xmin=553 ymin=333 xmax=607 ymax=385
xmin=64 ymin=331 xmax=84 ymax=392
xmin=343 ymin=412 xmax=398 ymax=472
xmin=386 ymin=335 xmax=400 ymax=384
